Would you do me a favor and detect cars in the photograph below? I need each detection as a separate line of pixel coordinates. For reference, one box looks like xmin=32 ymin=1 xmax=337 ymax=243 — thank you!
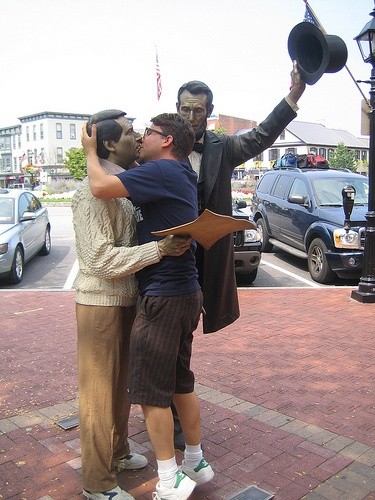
xmin=0 ymin=188 xmax=52 ymax=284
xmin=232 ymin=200 xmax=263 ymax=285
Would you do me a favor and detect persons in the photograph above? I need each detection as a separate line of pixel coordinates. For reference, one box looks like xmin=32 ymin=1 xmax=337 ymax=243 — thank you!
xmin=81 ymin=113 xmax=215 ymax=500
xmin=69 ymin=110 xmax=192 ymax=500
xmin=171 ymin=60 xmax=307 ymax=449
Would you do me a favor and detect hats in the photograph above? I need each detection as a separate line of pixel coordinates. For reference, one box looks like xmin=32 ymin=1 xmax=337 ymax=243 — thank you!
xmin=287 ymin=22 xmax=348 ymax=86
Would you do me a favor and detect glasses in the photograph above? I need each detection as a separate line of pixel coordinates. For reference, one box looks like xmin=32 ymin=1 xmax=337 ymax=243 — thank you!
xmin=143 ymin=128 xmax=179 ymax=147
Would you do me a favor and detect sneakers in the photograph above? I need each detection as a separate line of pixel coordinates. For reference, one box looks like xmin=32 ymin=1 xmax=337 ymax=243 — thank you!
xmin=111 ymin=454 xmax=148 ymax=470
xmin=180 ymin=457 xmax=215 ymax=487
xmin=82 ymin=485 xmax=135 ymax=500
xmin=152 ymin=470 xmax=196 ymax=500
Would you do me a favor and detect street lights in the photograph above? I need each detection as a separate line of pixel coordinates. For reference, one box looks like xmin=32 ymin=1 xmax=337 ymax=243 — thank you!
xmin=352 ymin=6 xmax=375 ymax=304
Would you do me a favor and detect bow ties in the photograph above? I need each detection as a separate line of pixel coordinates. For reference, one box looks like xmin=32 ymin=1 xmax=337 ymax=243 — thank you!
xmin=192 ymin=142 xmax=204 ymax=153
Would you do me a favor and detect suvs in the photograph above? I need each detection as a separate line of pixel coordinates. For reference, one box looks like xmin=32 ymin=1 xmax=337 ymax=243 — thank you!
xmin=250 ymin=153 xmax=369 ymax=285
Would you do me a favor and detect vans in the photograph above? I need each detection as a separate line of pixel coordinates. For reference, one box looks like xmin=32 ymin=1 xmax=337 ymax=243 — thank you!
xmin=7 ymin=182 xmax=33 ymax=191
